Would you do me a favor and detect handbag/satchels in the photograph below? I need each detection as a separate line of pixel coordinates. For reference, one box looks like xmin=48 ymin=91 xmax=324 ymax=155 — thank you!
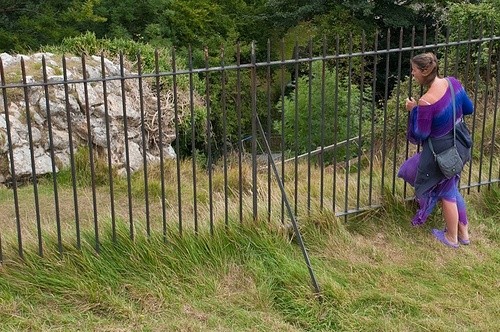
xmin=435 ymin=148 xmax=464 ymax=180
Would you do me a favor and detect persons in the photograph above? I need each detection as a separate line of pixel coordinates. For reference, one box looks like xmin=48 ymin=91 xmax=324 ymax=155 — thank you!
xmin=398 ymin=51 xmax=474 ymax=248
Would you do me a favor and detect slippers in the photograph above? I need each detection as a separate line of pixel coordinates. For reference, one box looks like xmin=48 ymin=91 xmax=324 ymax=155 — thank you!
xmin=445 ymin=227 xmax=470 ymax=245
xmin=431 ymin=228 xmax=459 ymax=248
